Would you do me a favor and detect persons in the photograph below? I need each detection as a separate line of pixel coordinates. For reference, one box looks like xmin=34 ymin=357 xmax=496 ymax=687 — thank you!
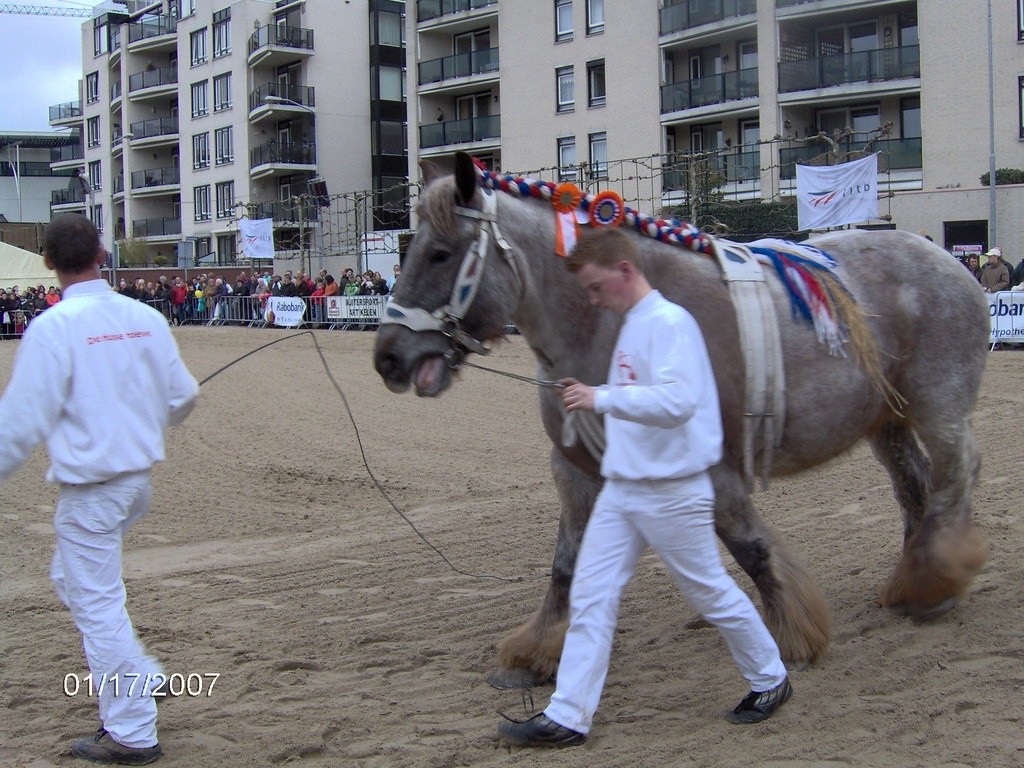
xmin=920 ymin=228 xmax=1024 ymax=293
xmin=0 ymin=274 xmax=205 ymax=340
xmin=199 ymin=264 xmax=401 ymax=331
xmin=496 ymin=230 xmax=793 ymax=749
xmin=0 ymin=213 xmax=200 ymax=766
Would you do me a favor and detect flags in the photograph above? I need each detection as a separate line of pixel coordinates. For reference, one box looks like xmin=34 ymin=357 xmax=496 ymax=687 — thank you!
xmin=796 ymin=152 xmax=878 ymax=232
xmin=236 ymin=218 xmax=274 ymax=259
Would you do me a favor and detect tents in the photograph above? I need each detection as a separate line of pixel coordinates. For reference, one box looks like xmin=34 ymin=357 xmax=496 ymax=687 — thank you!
xmin=0 ymin=241 xmax=60 ymax=296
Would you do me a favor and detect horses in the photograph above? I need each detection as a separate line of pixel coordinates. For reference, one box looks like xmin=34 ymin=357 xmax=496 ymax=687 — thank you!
xmin=371 ymin=147 xmax=990 ymax=692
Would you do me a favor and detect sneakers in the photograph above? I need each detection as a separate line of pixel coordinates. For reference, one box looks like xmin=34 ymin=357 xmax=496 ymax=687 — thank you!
xmin=497 ymin=710 xmax=586 ymax=749
xmin=725 ymin=678 xmax=794 ymax=723
xmin=70 ymin=726 xmax=162 ymax=766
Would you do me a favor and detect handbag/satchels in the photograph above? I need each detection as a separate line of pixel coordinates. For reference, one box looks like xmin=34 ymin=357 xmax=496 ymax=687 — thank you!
xmin=3 ymin=311 xmax=11 ymax=324
